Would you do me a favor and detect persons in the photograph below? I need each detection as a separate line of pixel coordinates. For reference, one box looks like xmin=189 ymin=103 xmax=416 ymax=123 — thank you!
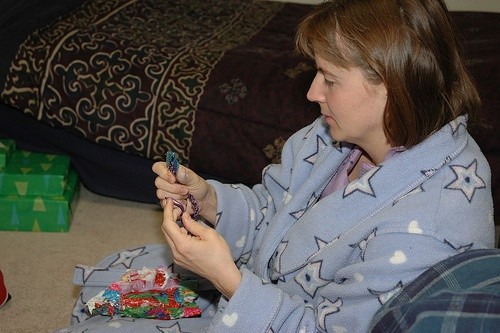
xmin=59 ymin=0 xmax=494 ymax=333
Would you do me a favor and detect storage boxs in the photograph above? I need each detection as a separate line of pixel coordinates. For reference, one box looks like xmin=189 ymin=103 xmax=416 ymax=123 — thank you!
xmin=0 ymin=172 xmax=81 ymax=233
xmin=0 ymin=150 xmax=72 ymax=197
xmin=0 ymin=138 xmax=16 ymax=169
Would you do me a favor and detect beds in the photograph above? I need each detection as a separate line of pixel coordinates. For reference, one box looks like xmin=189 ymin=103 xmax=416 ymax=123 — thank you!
xmin=0 ymin=0 xmax=500 ymax=225
